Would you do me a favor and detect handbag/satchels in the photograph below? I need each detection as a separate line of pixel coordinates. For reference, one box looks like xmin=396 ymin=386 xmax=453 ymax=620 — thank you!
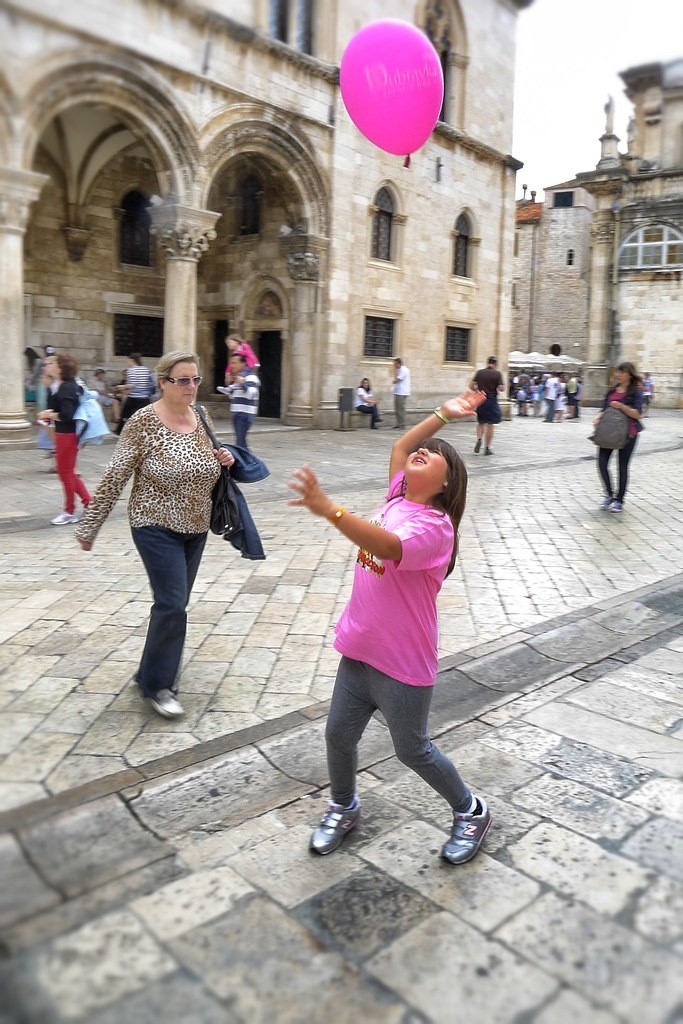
xmin=194 ymin=404 xmax=240 ymax=535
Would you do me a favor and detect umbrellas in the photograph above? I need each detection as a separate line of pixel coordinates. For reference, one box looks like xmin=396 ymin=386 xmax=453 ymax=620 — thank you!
xmin=507 ymin=351 xmax=587 ymax=367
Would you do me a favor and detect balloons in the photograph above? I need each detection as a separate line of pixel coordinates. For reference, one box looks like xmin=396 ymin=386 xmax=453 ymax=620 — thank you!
xmin=340 ymin=20 xmax=445 ymax=170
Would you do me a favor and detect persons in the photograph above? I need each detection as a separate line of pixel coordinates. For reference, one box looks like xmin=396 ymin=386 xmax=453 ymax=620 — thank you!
xmin=352 ymin=379 xmax=383 ymax=430
xmin=594 ymin=362 xmax=644 ymax=514
xmin=507 ymin=369 xmax=580 ymax=423
xmin=35 ymin=354 xmax=92 ymax=525
xmin=216 ymin=334 xmax=261 ymax=450
xmin=71 ymin=350 xmax=235 ymax=718
xmin=286 ymin=391 xmax=492 ymax=864
xmin=469 ymin=356 xmax=505 ymax=455
xmin=24 ymin=346 xmax=162 ymax=459
xmin=393 ymin=358 xmax=411 ymax=430
xmin=636 ymin=372 xmax=653 ymax=418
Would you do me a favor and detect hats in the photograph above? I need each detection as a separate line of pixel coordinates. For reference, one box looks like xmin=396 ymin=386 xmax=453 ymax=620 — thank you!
xmin=93 ymin=368 xmax=105 ymax=377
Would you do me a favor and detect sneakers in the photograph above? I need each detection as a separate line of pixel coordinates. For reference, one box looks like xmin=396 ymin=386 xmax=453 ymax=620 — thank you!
xmin=309 ymin=794 xmax=361 ymax=855
xmin=438 ymin=795 xmax=493 ymax=865
xmin=49 ymin=512 xmax=78 ymax=525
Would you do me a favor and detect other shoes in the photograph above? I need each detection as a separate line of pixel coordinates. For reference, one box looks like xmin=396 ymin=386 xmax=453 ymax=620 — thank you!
xmin=600 ymin=497 xmax=613 ymax=508
xmin=375 ymin=419 xmax=383 ymax=422
xmin=394 ymin=425 xmax=405 ymax=429
xmin=474 ymin=438 xmax=482 ymax=453
xmin=229 ymin=385 xmax=242 ymax=390
xmin=132 ymin=671 xmax=185 ymax=719
xmin=485 ymin=447 xmax=494 ymax=456
xmin=112 ymin=419 xmax=120 ymax=423
xmin=217 ymin=386 xmax=232 ymax=395
xmin=644 ymin=416 xmax=649 ymax=418
xmin=609 ymin=502 xmax=622 ymax=513
xmin=371 ymin=427 xmax=378 ymax=429
xmin=515 ymin=413 xmax=579 ymax=423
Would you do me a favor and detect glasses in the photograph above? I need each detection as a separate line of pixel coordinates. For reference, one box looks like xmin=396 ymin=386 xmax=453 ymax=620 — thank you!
xmin=165 ymin=376 xmax=203 ymax=386
xmin=41 ymin=362 xmax=53 ymax=367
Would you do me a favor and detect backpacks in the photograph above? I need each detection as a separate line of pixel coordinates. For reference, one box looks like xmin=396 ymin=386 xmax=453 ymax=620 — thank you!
xmin=594 ymin=407 xmax=628 ymax=449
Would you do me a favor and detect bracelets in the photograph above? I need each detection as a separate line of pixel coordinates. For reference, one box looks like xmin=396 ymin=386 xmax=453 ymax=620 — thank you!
xmin=331 ymin=507 xmax=344 ymax=523
xmin=434 ymin=407 xmax=450 ymax=423
